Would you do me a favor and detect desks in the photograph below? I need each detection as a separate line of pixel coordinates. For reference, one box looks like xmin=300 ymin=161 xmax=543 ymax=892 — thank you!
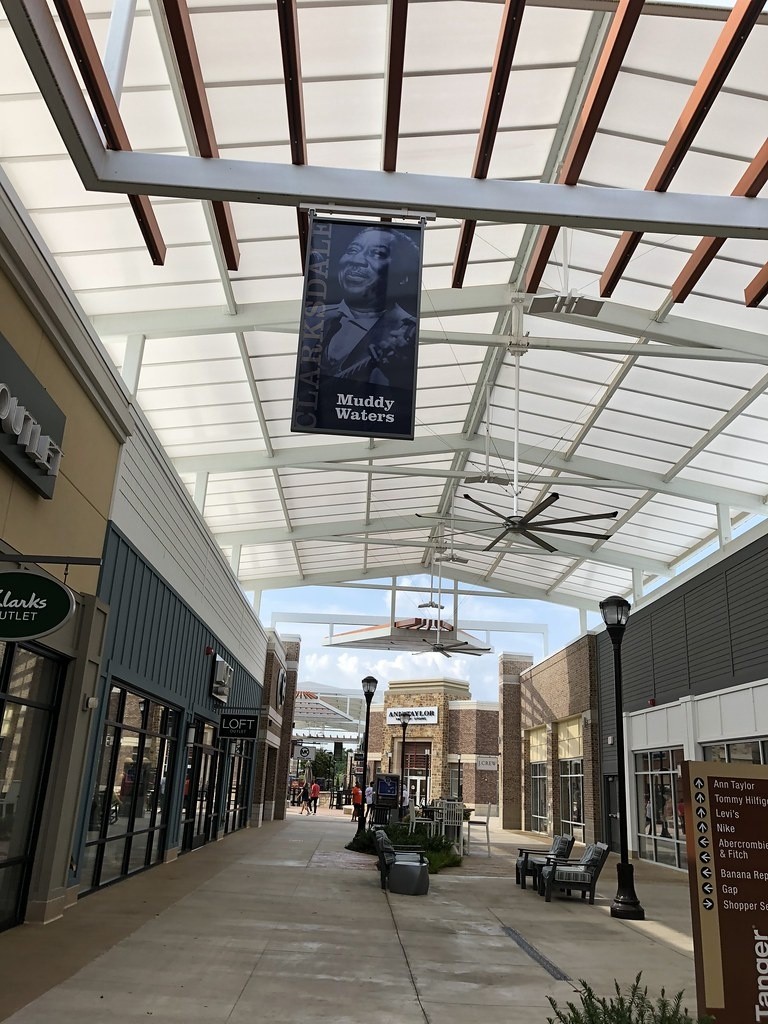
xmin=530 ymin=860 xmax=572 ymax=896
xmin=392 ymin=860 xmax=429 ymax=895
xmin=429 ymin=808 xmax=476 ymax=842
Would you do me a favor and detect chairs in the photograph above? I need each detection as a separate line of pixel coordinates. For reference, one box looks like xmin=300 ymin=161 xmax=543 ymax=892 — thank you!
xmin=368 ymin=798 xmax=492 ymax=858
xmin=515 ymin=832 xmax=575 ymax=888
xmin=540 ymin=842 xmax=611 ymax=903
xmin=372 ymin=827 xmax=431 ymax=891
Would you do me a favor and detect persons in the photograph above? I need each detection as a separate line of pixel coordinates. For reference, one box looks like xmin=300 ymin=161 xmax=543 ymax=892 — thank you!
xmin=645 ymin=801 xmax=652 ymax=836
xmin=113 ymin=756 xmax=152 ymax=819
xmin=350 ymin=782 xmax=375 ymax=824
xmin=400 ymin=784 xmax=410 ymax=818
xmin=677 ymin=798 xmax=686 ymax=837
xmin=303 ymin=228 xmax=419 ymax=389
xmin=296 ymin=779 xmax=320 ymax=816
xmin=157 ymin=770 xmax=190 ymax=813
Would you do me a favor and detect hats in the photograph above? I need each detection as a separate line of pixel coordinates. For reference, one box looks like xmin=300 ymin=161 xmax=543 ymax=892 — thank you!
xmin=402 ymin=785 xmax=406 ymax=789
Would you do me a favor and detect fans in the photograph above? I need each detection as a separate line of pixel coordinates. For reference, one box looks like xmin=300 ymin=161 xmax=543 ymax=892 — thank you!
xmin=411 ymin=548 xmax=490 ymax=658
xmin=413 ymin=344 xmax=617 ymax=553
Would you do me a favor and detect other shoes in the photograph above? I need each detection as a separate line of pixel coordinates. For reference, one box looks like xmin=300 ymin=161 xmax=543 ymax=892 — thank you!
xmin=310 ymin=811 xmax=313 ymax=814
xmin=351 ymin=819 xmax=358 ymax=822
xmin=306 ymin=812 xmax=311 ymax=816
xmin=312 ymin=813 xmax=316 ymax=815
xmin=299 ymin=812 xmax=302 ymax=814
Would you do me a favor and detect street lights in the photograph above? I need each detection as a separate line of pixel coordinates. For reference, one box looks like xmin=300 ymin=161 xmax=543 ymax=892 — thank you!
xmin=458 ymin=753 xmax=461 ymax=802
xmin=597 ymin=596 xmax=644 ymax=919
xmin=348 ymin=752 xmax=353 ymax=790
xmin=328 ymin=751 xmax=332 ymax=790
xmin=425 ymin=748 xmax=430 ymax=806
xmin=351 ymin=677 xmax=377 ymax=847
xmin=397 ymin=713 xmax=411 ymax=815
